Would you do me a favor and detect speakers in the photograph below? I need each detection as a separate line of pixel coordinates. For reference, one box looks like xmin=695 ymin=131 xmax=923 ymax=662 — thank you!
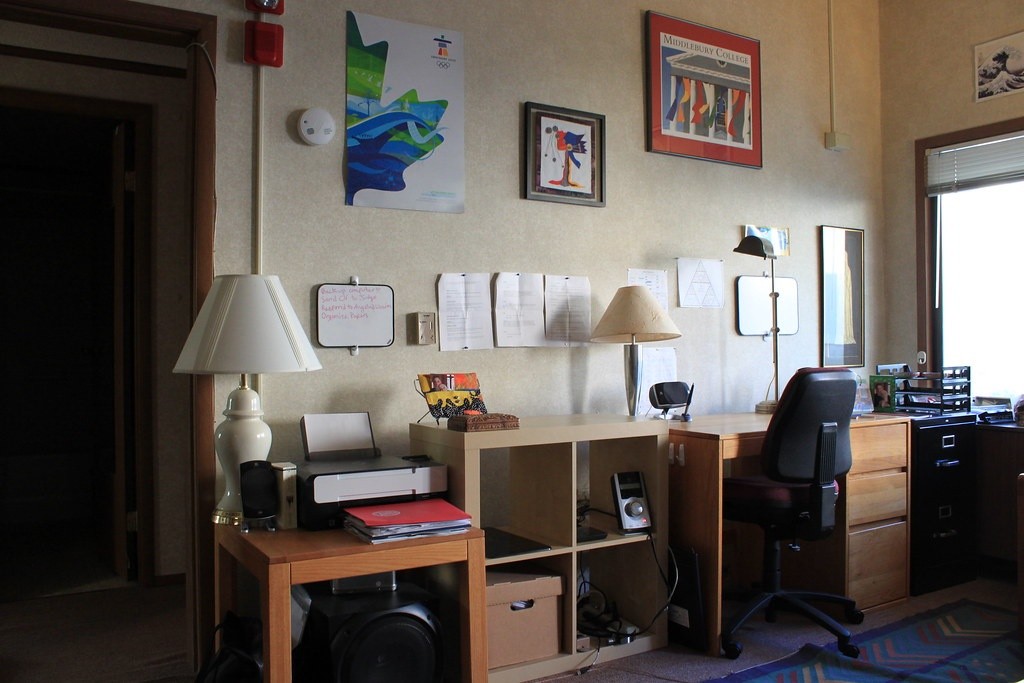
xmin=292 ymin=572 xmax=447 ymax=683
xmin=239 ymin=460 xmax=279 ymax=532
xmin=649 ymin=382 xmax=691 ymax=420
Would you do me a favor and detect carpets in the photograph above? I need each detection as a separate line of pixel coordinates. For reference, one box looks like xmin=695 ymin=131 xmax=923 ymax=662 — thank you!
xmin=700 ymin=597 xmax=1024 ymax=683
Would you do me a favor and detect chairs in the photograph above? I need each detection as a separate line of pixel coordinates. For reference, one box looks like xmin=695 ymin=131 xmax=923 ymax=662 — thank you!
xmin=723 ymin=367 xmax=865 ymax=661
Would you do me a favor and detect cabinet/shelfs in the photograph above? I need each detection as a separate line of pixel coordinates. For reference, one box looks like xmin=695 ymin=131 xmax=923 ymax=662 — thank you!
xmin=670 ymin=408 xmax=915 ymax=655
xmin=876 ymin=363 xmax=971 ymax=416
xmin=408 ymin=414 xmax=671 ymax=683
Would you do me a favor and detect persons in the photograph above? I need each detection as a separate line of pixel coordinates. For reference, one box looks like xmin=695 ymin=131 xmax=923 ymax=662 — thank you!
xmin=431 ymin=376 xmax=450 ymax=392
xmin=875 ymin=384 xmax=889 ymax=407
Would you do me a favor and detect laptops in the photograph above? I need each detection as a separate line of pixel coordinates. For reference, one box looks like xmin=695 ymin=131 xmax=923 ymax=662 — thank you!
xmin=331 ymin=578 xmax=397 ymax=594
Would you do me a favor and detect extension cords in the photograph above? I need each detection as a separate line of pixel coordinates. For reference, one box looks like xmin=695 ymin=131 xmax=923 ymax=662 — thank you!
xmin=586 ymin=604 xmax=639 ymax=636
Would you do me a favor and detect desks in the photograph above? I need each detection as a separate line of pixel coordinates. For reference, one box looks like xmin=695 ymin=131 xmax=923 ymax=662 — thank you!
xmin=977 ymin=420 xmax=1024 ymax=585
xmin=213 ymin=499 xmax=487 ymax=683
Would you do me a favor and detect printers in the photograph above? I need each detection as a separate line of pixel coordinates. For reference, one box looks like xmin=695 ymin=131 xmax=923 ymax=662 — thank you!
xmin=294 ymin=413 xmax=448 ymax=532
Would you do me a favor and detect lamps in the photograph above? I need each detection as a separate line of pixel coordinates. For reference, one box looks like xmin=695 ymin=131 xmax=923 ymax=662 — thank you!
xmin=174 ymin=271 xmax=322 ymax=528
xmin=734 ymin=236 xmax=783 ymax=415
xmin=588 ymin=283 xmax=682 ymax=417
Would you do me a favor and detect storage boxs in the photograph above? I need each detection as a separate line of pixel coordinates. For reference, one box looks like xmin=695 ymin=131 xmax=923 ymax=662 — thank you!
xmin=485 ymin=571 xmax=561 ymax=671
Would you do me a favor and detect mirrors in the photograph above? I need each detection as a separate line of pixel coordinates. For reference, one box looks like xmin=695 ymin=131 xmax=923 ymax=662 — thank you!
xmin=821 ymin=224 xmax=866 ymax=369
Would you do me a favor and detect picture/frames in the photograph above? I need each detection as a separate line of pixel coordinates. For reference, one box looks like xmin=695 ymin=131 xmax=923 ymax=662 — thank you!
xmin=646 ymin=8 xmax=763 ymax=171
xmin=869 ymin=374 xmax=895 ymax=414
xmin=524 ymin=100 xmax=608 ymax=210
xmin=974 ymin=29 xmax=1024 ymax=103
xmin=744 ymin=224 xmax=791 ymax=258
